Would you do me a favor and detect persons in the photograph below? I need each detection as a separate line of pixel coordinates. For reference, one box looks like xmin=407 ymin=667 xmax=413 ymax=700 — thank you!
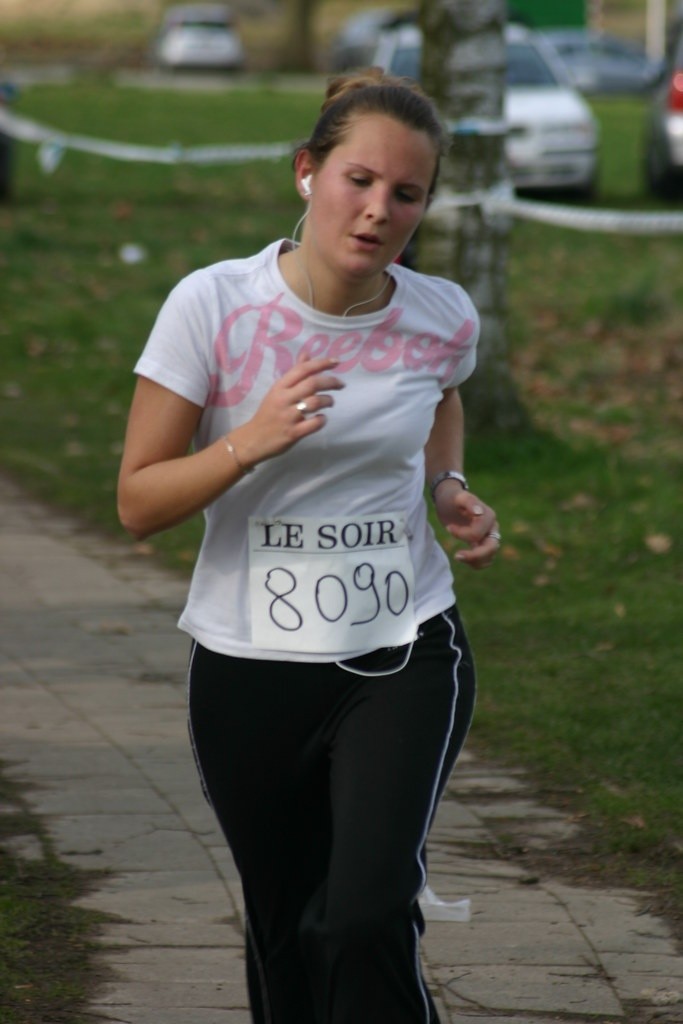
xmin=116 ymin=68 xmax=503 ymax=1024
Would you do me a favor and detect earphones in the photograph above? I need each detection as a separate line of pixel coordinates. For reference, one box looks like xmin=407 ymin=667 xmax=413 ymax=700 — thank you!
xmin=300 ymin=175 xmax=313 ymax=196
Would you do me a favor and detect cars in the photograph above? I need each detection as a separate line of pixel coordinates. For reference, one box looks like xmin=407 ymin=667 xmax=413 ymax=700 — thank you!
xmin=536 ymin=28 xmax=656 ymax=94
xmin=640 ymin=21 xmax=683 ymax=203
xmin=326 ymin=6 xmax=604 ymax=203
xmin=155 ymin=3 xmax=244 ymax=74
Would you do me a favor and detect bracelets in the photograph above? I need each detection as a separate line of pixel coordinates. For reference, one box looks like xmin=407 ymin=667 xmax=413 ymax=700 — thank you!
xmin=220 ymin=434 xmax=257 ymax=475
xmin=431 ymin=470 xmax=469 ymax=501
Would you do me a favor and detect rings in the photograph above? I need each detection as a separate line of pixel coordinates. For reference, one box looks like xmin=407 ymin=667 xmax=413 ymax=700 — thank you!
xmin=488 ymin=531 xmax=502 ymax=545
xmin=297 ymin=402 xmax=308 ymax=413
